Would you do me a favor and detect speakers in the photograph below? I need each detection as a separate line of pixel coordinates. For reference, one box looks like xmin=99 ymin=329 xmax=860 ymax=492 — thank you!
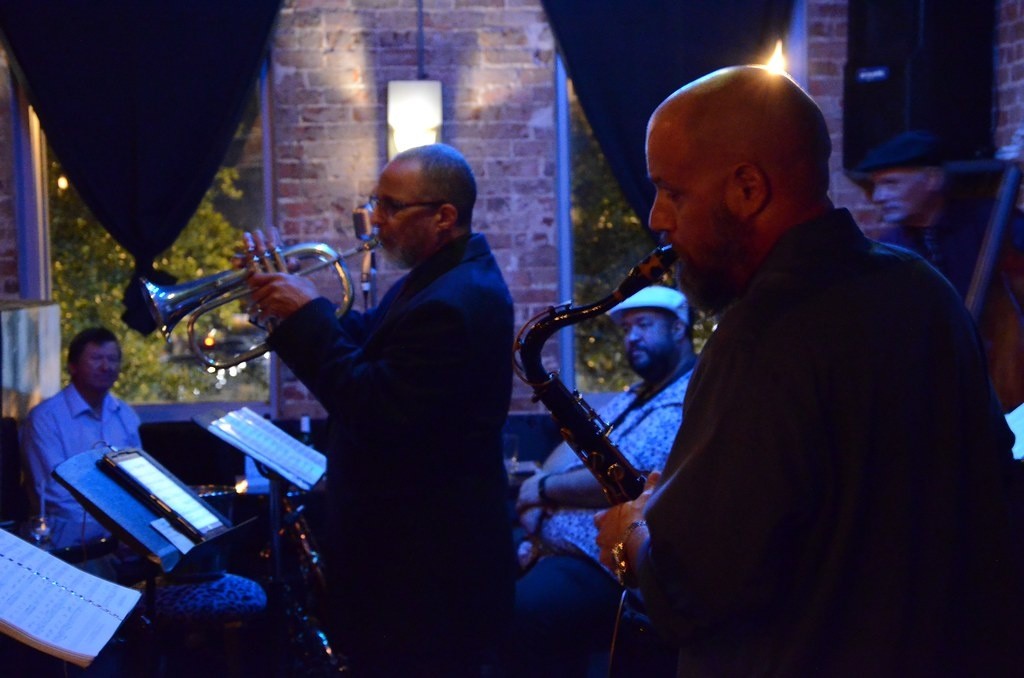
xmin=842 ymin=0 xmax=1000 ymax=171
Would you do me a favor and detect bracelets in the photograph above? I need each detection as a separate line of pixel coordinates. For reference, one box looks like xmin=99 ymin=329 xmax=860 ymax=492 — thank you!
xmin=539 ymin=473 xmax=561 ymax=506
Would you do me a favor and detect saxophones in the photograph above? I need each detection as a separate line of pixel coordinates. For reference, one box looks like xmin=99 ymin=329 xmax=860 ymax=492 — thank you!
xmin=512 ymin=240 xmax=677 ymax=508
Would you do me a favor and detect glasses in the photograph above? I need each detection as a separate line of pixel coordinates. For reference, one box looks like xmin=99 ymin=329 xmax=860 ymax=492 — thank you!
xmin=369 ymin=194 xmax=441 ymax=217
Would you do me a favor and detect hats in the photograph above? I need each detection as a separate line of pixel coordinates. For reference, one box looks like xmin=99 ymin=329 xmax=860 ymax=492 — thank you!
xmin=605 ymin=286 xmax=689 ymax=325
xmin=856 ymin=132 xmax=942 ymax=171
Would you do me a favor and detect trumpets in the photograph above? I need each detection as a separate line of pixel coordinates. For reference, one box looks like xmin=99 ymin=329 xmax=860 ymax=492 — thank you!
xmin=141 ymin=234 xmax=379 ymax=368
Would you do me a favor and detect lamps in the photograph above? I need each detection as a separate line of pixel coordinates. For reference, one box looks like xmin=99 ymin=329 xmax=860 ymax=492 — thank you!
xmin=387 ymin=79 xmax=443 ymax=161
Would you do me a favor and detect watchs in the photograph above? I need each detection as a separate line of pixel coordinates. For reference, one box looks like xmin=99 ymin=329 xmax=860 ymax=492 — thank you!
xmin=610 ymin=520 xmax=647 ymax=589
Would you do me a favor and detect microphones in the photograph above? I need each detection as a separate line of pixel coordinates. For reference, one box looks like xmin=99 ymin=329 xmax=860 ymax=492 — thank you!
xmin=353 ymin=203 xmax=376 ymax=293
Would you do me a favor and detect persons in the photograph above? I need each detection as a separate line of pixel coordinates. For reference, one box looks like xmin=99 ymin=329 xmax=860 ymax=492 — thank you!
xmin=15 ymin=325 xmax=141 ymax=678
xmin=510 ymin=286 xmax=700 ymax=678
xmin=855 ymin=130 xmax=1024 ymax=414
xmin=246 ymin=146 xmax=512 ymax=678
xmin=591 ymin=62 xmax=1024 ymax=678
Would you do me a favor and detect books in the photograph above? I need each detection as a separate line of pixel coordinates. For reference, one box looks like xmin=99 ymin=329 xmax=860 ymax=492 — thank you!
xmin=0 ymin=526 xmax=140 ymax=666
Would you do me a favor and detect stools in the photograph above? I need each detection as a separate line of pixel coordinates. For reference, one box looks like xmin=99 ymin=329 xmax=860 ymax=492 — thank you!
xmin=126 ymin=573 xmax=266 ymax=678
